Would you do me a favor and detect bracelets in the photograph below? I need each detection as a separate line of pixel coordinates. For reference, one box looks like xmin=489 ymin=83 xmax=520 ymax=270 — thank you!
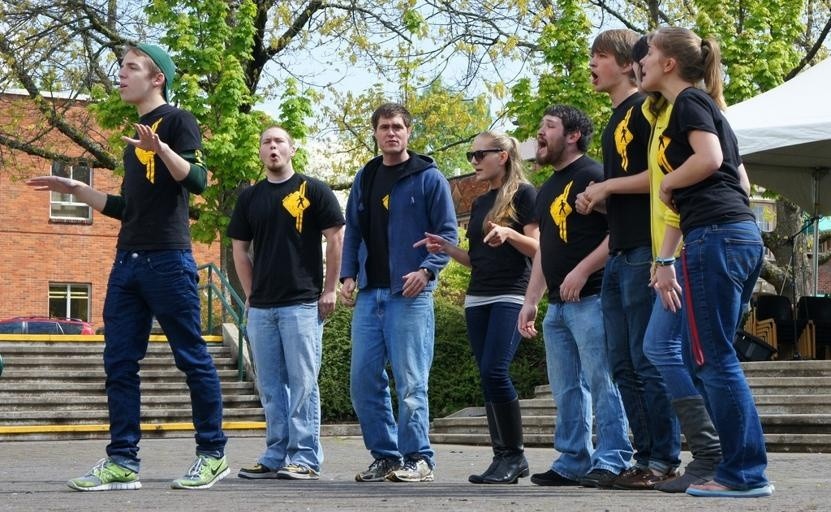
xmin=422 ymin=267 xmax=432 ymax=278
xmin=654 ymin=256 xmax=675 ymax=265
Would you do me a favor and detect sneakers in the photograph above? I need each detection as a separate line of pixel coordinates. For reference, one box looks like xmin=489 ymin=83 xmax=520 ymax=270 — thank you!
xmin=528 ymin=470 xmax=579 ymax=487
xmin=237 ymin=461 xmax=283 ymax=480
xmin=170 ymin=450 xmax=231 ymax=491
xmin=274 ymin=462 xmax=321 ymax=482
xmin=579 ymin=465 xmax=617 ymax=488
xmin=385 ymin=452 xmax=436 ymax=482
xmin=356 ymin=452 xmax=404 ymax=483
xmin=67 ymin=459 xmax=143 ymax=491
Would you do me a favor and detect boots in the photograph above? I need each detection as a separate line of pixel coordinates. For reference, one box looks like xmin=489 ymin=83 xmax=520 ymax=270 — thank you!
xmin=469 ymin=396 xmax=530 ymax=484
xmin=654 ymin=395 xmax=723 ymax=493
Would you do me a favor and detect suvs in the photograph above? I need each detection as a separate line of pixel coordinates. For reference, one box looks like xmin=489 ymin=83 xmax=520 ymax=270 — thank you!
xmin=1 ymin=316 xmax=97 ymax=334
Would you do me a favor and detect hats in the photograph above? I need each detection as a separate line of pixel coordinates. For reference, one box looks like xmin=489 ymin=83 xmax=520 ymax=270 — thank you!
xmin=137 ymin=44 xmax=176 ymax=106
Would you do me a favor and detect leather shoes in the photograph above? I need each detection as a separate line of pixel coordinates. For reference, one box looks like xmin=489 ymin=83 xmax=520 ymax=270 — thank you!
xmin=612 ymin=462 xmax=680 ymax=490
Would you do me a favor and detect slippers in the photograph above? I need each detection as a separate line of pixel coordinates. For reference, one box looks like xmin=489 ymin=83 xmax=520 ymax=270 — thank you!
xmin=685 ymin=478 xmax=777 ymax=497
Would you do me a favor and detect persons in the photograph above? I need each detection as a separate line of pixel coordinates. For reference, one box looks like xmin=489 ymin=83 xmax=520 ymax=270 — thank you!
xmin=574 ymin=27 xmax=681 ymax=490
xmin=339 ymin=104 xmax=459 ymax=486
xmin=629 ymin=34 xmax=724 ymax=493
xmin=640 ymin=26 xmax=775 ymax=497
xmin=25 ymin=46 xmax=231 ymax=494
xmin=515 ymin=104 xmax=636 ymax=489
xmin=228 ymin=127 xmax=345 ymax=479
xmin=412 ymin=130 xmax=539 ymax=486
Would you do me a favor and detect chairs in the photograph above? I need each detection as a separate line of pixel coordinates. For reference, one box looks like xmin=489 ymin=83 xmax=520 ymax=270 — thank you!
xmin=744 ymin=290 xmax=830 ymax=360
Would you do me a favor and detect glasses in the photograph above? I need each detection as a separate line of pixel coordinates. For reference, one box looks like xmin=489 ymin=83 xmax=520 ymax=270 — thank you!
xmin=465 ymin=149 xmax=503 ymax=163
